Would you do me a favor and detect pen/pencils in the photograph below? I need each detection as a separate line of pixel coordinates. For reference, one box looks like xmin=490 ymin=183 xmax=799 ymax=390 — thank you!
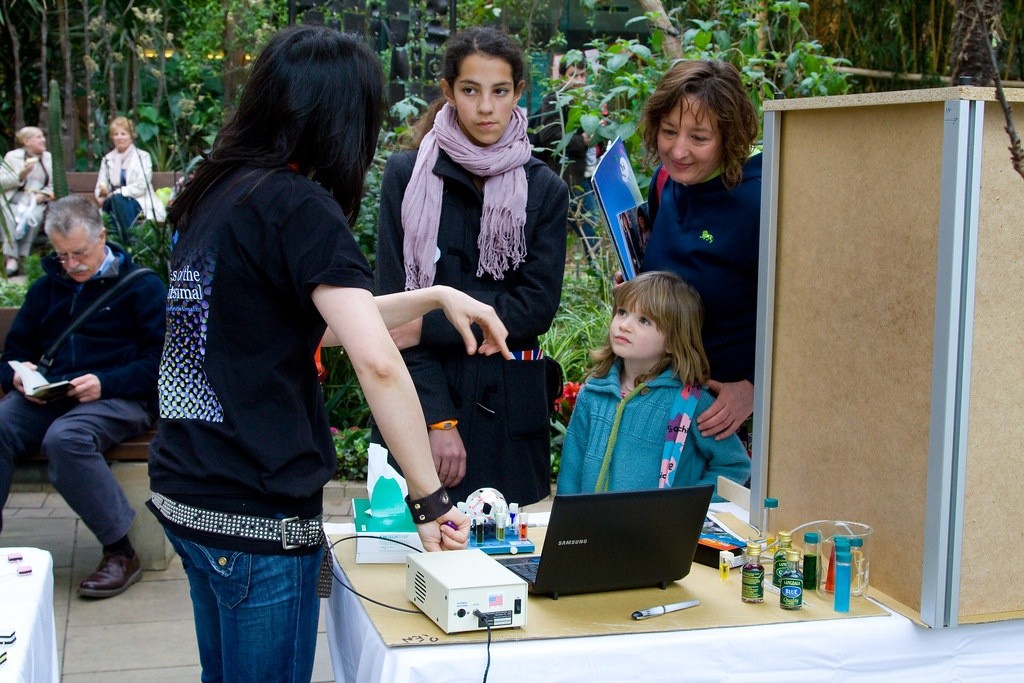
xmin=33 ymin=384 xmax=49 ymax=391
xmin=631 ymin=600 xmax=701 ymax=620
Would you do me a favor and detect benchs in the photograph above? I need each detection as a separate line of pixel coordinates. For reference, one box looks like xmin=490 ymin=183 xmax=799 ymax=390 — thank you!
xmin=40 ymin=173 xmax=184 ymax=228
xmin=0 ymin=306 xmax=177 ymax=571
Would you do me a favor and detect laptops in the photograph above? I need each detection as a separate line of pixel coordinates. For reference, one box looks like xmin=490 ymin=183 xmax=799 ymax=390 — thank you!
xmin=496 ymin=484 xmax=714 ymax=599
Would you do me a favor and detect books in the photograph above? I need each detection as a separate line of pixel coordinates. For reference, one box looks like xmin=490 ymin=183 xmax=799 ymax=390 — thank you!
xmin=590 ymin=135 xmax=648 ymax=281
xmin=8 ymin=361 xmax=76 ymax=402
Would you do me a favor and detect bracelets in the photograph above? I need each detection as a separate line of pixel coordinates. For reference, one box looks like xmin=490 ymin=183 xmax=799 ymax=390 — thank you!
xmin=404 ymin=484 xmax=454 ymax=524
xmin=428 ymin=420 xmax=458 ymax=430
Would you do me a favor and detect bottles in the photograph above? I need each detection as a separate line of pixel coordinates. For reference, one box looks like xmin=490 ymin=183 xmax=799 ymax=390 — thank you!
xmin=741 ymin=541 xmax=765 ymax=603
xmin=823 ymin=534 xmax=872 ymax=613
xmin=803 ymin=532 xmax=822 ymax=591
xmin=759 ymin=499 xmax=786 ymax=556
xmin=780 ymin=546 xmax=804 ymax=611
xmin=772 ymin=530 xmax=795 ymax=588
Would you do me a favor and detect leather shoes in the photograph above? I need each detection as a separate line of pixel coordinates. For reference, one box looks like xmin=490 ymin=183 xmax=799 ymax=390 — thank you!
xmin=78 ymin=552 xmax=142 ymax=598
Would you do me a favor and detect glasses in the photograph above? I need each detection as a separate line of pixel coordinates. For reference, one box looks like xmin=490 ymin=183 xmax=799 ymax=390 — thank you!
xmin=55 ymin=252 xmax=88 ymax=263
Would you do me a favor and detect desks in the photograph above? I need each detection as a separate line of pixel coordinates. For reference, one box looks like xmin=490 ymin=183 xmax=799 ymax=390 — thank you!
xmin=0 ymin=547 xmax=60 ymax=683
xmin=322 ymin=501 xmax=1024 ymax=683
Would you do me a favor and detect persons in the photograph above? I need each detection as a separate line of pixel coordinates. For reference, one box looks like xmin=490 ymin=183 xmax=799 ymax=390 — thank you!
xmin=0 ymin=126 xmax=55 ymax=278
xmin=376 ymin=26 xmax=569 ymax=505
xmin=555 ymin=272 xmax=752 ymax=502
xmin=636 ymin=206 xmax=650 ymax=249
xmin=523 ymin=53 xmax=614 ymax=301
xmin=622 ymin=211 xmax=645 ymax=273
xmin=94 ymin=117 xmax=152 ymax=246
xmin=143 ymin=26 xmax=511 ymax=683
xmin=611 ymin=61 xmax=762 ymax=489
xmin=0 ymin=194 xmax=165 ymax=598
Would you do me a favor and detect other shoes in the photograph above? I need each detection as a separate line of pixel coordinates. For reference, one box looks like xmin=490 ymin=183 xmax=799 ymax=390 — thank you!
xmin=5 ymin=264 xmax=20 ymax=276
